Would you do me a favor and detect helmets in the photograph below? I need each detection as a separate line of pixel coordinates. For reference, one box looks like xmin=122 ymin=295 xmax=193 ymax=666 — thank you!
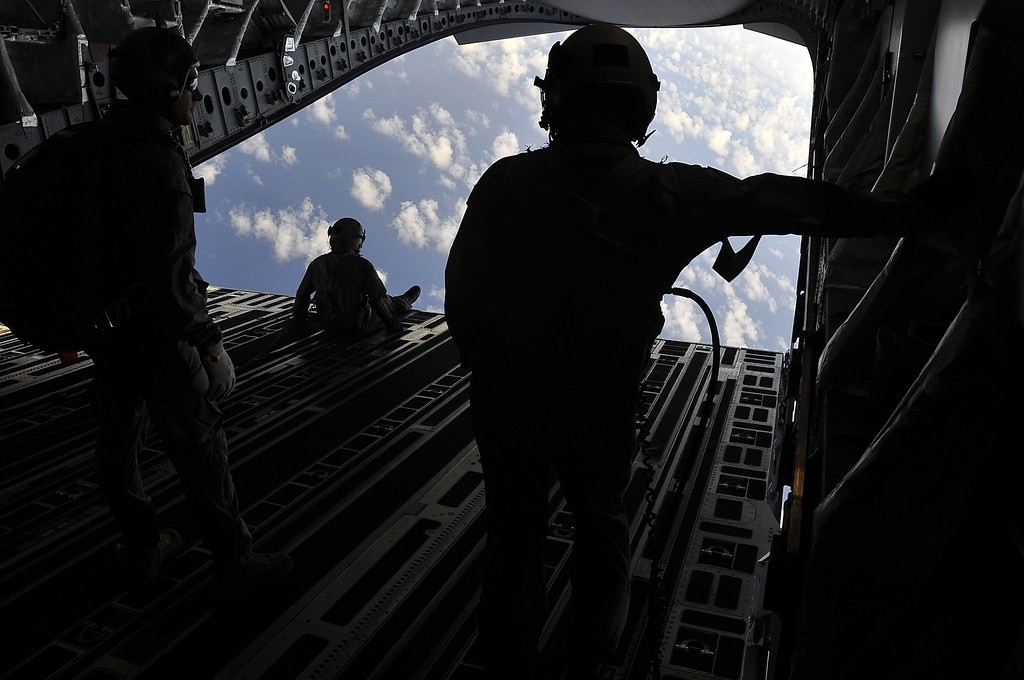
xmin=330 ymin=218 xmax=364 ymax=253
xmin=542 ymin=23 xmax=660 ymax=145
xmin=110 ymin=26 xmax=200 ymax=120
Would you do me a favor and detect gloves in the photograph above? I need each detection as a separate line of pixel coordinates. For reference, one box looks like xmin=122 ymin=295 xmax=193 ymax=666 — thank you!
xmin=292 ymin=295 xmax=307 ymax=313
xmin=195 ymin=323 xmax=236 ymax=403
xmin=375 ymin=303 xmax=396 ymax=330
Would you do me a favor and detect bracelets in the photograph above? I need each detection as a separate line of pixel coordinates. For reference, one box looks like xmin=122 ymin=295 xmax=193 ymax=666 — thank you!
xmin=202 ymin=350 xmax=224 ymax=365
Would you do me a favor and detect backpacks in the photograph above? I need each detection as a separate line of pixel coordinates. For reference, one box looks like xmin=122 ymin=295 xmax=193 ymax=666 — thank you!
xmin=0 ymin=120 xmax=191 ymax=349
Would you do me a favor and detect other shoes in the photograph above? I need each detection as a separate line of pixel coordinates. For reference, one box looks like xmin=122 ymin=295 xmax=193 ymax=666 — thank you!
xmin=403 ymin=286 xmax=420 ymax=303
xmin=128 ymin=529 xmax=181 ymax=576
xmin=215 ymin=550 xmax=294 ymax=598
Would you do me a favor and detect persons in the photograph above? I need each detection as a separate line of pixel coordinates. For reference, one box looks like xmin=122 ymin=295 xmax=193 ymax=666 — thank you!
xmin=292 ymin=218 xmax=421 ymax=336
xmin=445 ymin=23 xmax=936 ymax=680
xmin=89 ymin=27 xmax=293 ymax=590
